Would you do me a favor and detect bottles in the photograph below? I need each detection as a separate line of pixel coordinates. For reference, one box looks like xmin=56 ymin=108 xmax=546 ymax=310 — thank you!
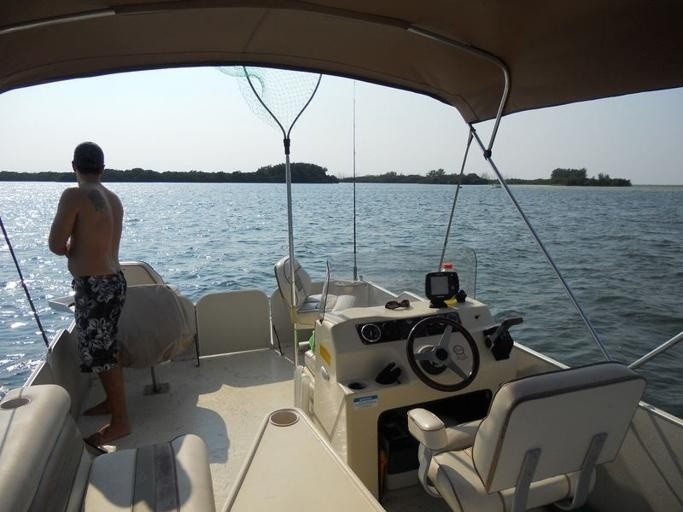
xmin=440 ymin=262 xmax=458 ymax=305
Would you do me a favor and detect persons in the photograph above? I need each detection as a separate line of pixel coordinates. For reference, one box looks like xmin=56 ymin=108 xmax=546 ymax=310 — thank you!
xmin=48 ymin=141 xmax=134 ymax=448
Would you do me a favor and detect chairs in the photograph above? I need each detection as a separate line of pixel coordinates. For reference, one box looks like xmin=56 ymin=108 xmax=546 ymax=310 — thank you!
xmin=0 ymin=381 xmax=216 ymax=512
xmin=275 ymin=254 xmax=356 ymax=368
xmin=117 ymin=257 xmax=199 ymax=391
xmin=407 ymin=360 xmax=647 ymax=512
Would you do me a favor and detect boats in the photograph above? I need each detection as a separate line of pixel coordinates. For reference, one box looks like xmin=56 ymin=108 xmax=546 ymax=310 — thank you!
xmin=0 ymin=2 xmax=682 ymax=512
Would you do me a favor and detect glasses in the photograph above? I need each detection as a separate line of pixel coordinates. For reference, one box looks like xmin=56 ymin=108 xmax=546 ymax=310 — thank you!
xmin=385 ymin=300 xmax=409 ymax=309
